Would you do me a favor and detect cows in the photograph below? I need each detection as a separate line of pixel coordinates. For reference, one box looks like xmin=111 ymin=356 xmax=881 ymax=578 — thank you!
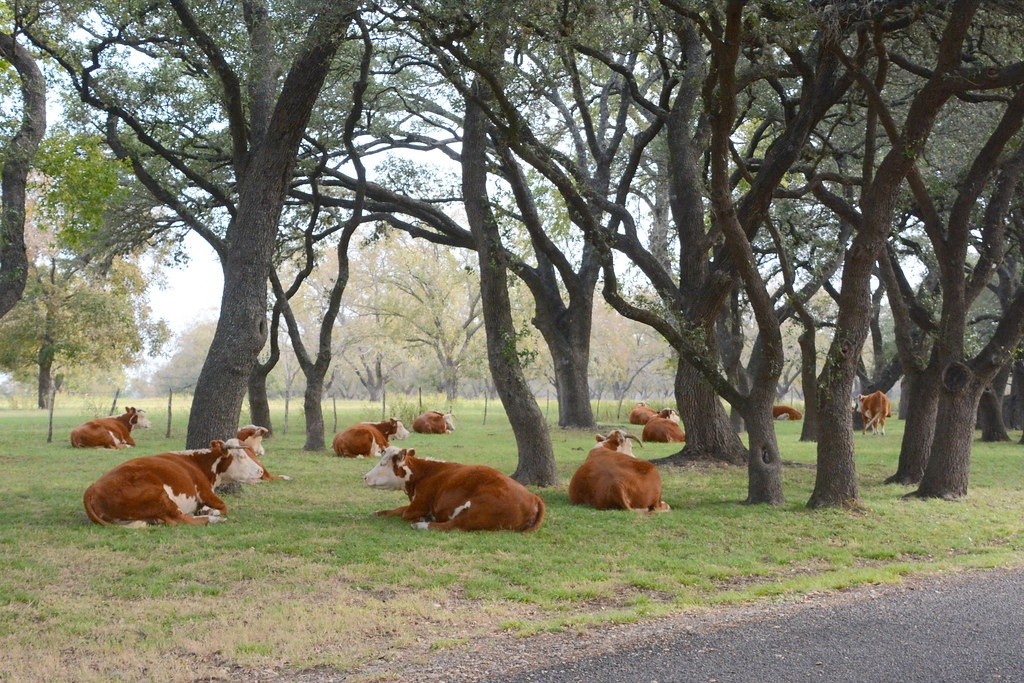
xmin=330 ymin=409 xmax=546 ymax=535
xmin=568 ymin=429 xmax=674 ymax=514
xmin=772 ymin=405 xmax=803 ymax=422
xmin=69 ymin=405 xmax=152 ymax=451
xmin=628 ymin=399 xmax=687 ymax=444
xmin=853 ymin=390 xmax=894 ymax=436
xmin=81 ymin=424 xmax=294 ymax=530
